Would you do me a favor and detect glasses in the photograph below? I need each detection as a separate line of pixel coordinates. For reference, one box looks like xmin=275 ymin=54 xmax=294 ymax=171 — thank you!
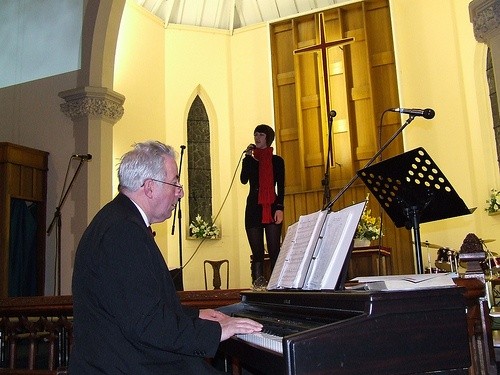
xmin=140 ymin=179 xmax=184 ymax=194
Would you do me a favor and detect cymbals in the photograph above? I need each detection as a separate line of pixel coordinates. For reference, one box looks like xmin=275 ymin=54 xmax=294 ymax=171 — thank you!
xmin=483 ymin=251 xmax=499 ymax=257
xmin=480 ymin=239 xmax=497 ymax=245
xmin=411 ymin=241 xmax=442 ymax=249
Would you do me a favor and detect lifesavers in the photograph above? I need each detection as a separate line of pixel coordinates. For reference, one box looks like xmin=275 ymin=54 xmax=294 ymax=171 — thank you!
xmin=434 ymin=246 xmax=457 ymax=273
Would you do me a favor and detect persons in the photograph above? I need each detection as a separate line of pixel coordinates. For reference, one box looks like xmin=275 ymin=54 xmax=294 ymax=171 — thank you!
xmin=459 ymin=233 xmax=491 ymax=314
xmin=240 ymin=125 xmax=285 ymax=290
xmin=66 ymin=141 xmax=263 ymax=375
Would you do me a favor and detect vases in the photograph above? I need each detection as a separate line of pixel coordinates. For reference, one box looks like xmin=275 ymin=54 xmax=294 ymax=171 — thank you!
xmin=353 ymin=238 xmax=371 ymax=247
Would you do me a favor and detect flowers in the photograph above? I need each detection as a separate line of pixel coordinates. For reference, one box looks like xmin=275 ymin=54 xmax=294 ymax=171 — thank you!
xmin=354 ymin=209 xmax=385 ymax=242
xmin=189 ymin=213 xmax=220 ymax=240
xmin=483 ymin=188 xmax=500 ymax=214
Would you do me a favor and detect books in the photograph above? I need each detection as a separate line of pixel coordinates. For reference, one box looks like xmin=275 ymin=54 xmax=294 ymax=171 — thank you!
xmin=265 ymin=201 xmax=367 ymax=292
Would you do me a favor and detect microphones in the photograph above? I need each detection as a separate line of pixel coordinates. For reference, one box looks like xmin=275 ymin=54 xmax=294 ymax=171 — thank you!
xmin=329 ymin=111 xmax=336 ymax=117
xmin=388 ymin=108 xmax=435 ymax=119
xmin=72 ymin=153 xmax=92 ymax=159
xmin=243 ymin=144 xmax=256 ymax=153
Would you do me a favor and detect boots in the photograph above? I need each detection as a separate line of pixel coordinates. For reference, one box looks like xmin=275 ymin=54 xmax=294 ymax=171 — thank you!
xmin=251 ymin=259 xmax=263 ymax=284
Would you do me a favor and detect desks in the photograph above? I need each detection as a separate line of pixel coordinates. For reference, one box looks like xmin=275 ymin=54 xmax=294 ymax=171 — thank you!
xmin=250 ymin=245 xmax=392 ymax=286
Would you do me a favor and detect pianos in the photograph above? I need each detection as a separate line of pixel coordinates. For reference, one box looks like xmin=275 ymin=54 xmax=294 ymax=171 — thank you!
xmin=214 ymin=286 xmax=475 ymax=375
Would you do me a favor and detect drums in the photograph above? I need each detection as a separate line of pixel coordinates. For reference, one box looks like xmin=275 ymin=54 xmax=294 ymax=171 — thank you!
xmin=485 ymin=257 xmax=500 ymax=275
